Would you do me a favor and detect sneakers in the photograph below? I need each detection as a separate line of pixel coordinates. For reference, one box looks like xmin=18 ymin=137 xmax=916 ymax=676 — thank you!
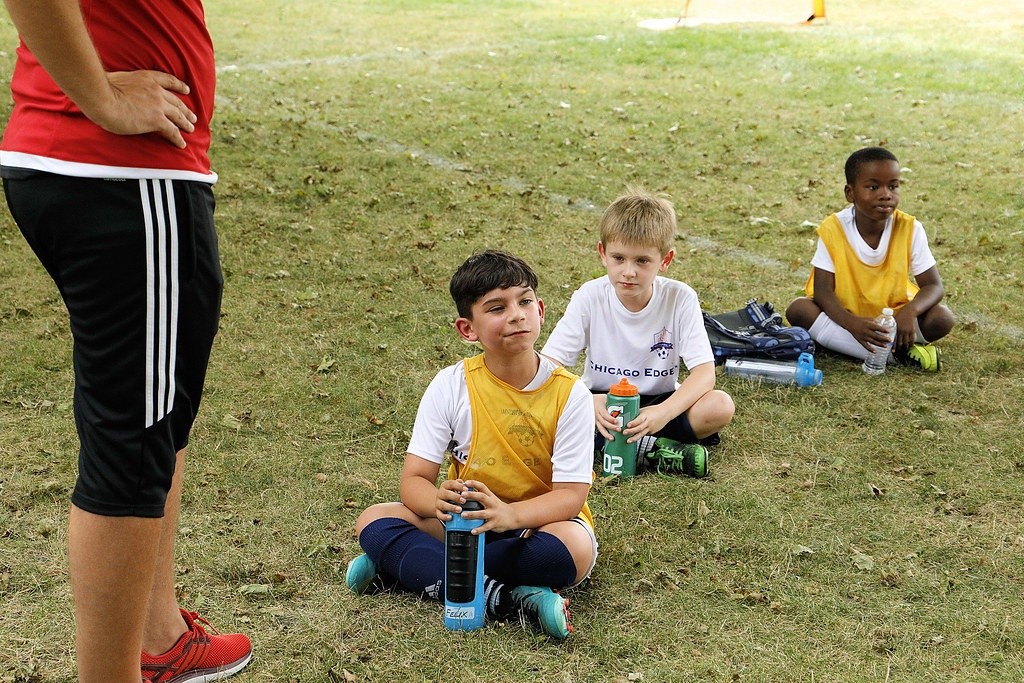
xmin=345 ymin=554 xmax=403 ymax=596
xmin=893 ymin=342 xmax=943 ymax=372
xmin=644 ymin=437 xmax=708 ymax=477
xmin=141 ymin=608 xmax=253 ymax=683
xmin=510 ymin=586 xmax=572 ymax=640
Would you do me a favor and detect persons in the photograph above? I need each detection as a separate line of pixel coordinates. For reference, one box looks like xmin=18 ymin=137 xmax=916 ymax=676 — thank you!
xmin=0 ymin=0 xmax=252 ymax=683
xmin=786 ymin=146 xmax=954 ymax=373
xmin=346 ymin=249 xmax=599 ymax=641
xmin=542 ymin=185 xmax=735 ymax=477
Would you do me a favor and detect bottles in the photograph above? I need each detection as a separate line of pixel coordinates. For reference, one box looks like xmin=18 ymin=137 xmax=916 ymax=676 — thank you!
xmin=724 ymin=352 xmax=822 ymax=386
xmin=861 ymin=307 xmax=897 ymax=375
xmin=444 ymin=481 xmax=484 ymax=631
xmin=603 ymin=376 xmax=640 ymax=478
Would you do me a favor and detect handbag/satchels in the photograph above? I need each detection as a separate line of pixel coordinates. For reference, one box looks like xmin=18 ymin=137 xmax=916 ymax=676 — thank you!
xmin=701 ymin=299 xmax=815 ymax=367
